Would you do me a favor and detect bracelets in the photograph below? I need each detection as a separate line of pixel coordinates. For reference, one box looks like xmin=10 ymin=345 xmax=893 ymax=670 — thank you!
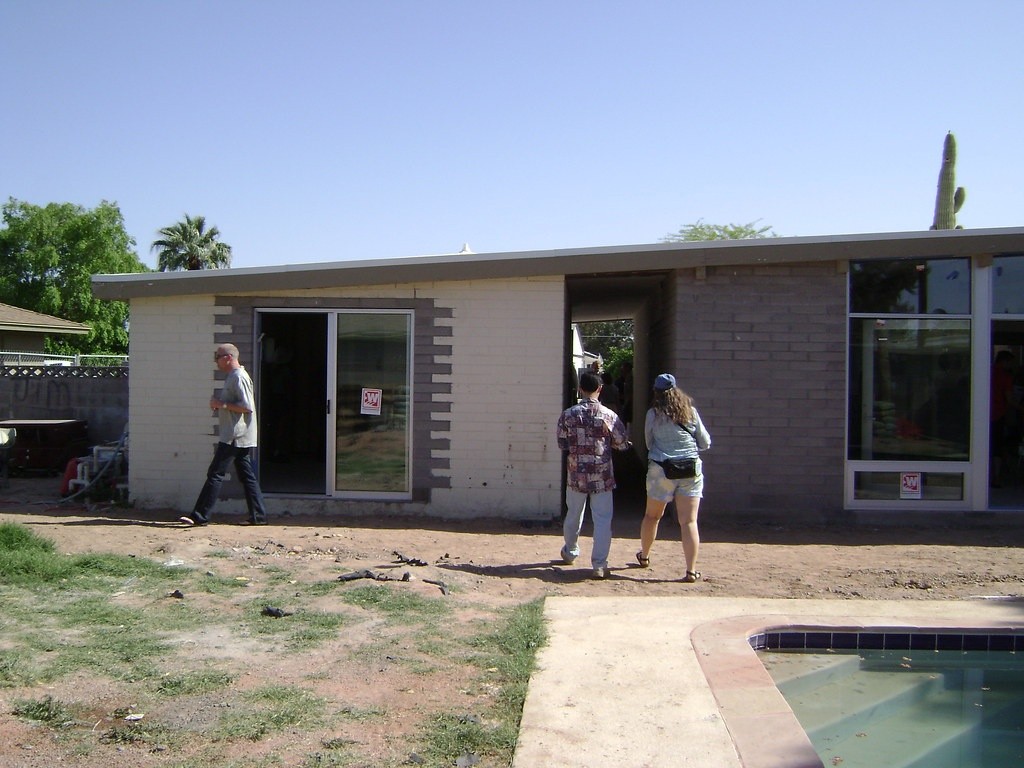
xmin=223 ymin=403 xmax=227 ymax=410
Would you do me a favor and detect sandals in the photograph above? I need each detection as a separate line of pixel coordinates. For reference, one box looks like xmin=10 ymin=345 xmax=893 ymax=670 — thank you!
xmin=686 ymin=571 xmax=701 ymax=582
xmin=636 ymin=551 xmax=650 ymax=567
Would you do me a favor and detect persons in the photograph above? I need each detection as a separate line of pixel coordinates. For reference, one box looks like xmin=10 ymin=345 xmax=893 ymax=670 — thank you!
xmin=590 ymin=361 xmax=604 ymax=376
xmin=572 ymin=363 xmax=579 ymax=405
xmin=178 ymin=343 xmax=268 ymax=525
xmin=637 ymin=374 xmax=713 ymax=583
xmin=930 ymin=350 xmax=1021 ymax=483
xmin=597 ymin=363 xmax=633 ymax=417
xmin=556 ymin=372 xmax=634 ymax=578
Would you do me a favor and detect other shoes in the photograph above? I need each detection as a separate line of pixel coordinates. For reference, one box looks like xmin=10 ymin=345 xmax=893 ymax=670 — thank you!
xmin=561 ymin=550 xmax=574 ymax=564
xmin=180 ymin=516 xmax=207 ymax=526
xmin=238 ymin=519 xmax=267 ymax=525
xmin=593 ymin=566 xmax=610 ymax=577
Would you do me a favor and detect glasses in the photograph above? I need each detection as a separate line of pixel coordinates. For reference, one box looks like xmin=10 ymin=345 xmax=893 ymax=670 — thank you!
xmin=216 ymin=354 xmax=232 ymax=359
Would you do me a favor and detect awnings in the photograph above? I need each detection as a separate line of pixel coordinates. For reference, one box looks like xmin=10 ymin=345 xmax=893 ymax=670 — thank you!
xmin=0 ymin=303 xmax=92 ymax=334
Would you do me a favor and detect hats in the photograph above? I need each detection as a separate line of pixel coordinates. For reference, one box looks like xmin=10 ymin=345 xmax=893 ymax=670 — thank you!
xmin=654 ymin=373 xmax=675 ymax=389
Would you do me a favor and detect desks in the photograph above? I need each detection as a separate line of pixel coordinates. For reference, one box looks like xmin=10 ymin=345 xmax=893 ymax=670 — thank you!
xmin=0 ymin=418 xmax=89 ymax=479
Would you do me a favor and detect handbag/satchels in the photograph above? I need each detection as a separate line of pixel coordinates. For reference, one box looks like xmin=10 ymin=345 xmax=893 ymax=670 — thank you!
xmin=653 ymin=458 xmax=696 ymax=479
